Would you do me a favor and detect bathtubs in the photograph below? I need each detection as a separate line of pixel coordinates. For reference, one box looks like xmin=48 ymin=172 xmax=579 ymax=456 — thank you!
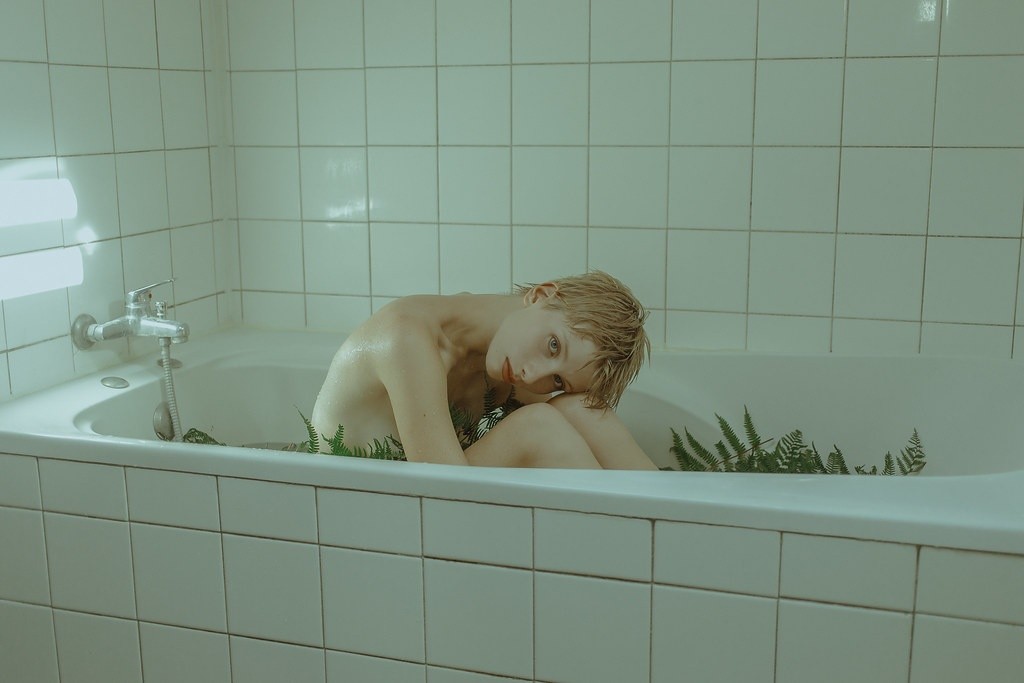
xmin=0 ymin=328 xmax=1021 ymax=681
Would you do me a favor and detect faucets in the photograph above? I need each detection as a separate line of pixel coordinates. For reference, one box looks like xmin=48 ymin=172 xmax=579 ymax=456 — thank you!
xmin=125 ymin=276 xmax=190 ymax=345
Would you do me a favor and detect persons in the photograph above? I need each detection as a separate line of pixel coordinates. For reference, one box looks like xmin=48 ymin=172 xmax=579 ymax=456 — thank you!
xmin=310 ymin=270 xmax=660 ymax=471
